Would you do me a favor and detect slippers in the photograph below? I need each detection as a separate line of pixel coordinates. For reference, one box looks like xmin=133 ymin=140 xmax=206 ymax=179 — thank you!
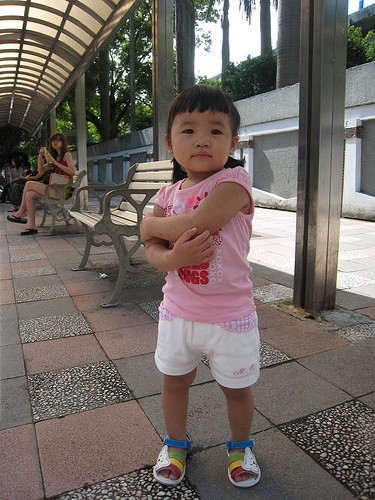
xmin=21 ymin=228 xmax=38 ymax=235
xmin=7 ymin=214 xmax=27 ymax=224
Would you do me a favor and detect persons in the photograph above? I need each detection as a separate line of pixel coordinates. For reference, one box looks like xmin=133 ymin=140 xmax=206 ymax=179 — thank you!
xmin=0 ymin=152 xmax=53 ymax=213
xmin=139 ymin=84 xmax=261 ymax=488
xmin=7 ymin=132 xmax=75 ymax=235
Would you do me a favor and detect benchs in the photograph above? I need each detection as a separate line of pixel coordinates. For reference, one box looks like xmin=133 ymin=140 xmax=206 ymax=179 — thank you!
xmin=67 ymin=161 xmax=174 ymax=308
xmin=37 ymin=170 xmax=87 ymax=236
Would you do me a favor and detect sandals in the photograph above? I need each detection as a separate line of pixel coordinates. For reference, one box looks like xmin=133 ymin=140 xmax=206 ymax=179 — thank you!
xmin=225 ymin=432 xmax=262 ymax=488
xmin=153 ymin=430 xmax=193 ymax=486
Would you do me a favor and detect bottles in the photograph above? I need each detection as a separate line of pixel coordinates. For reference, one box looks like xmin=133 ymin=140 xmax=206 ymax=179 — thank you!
xmin=43 ymin=148 xmax=54 ymax=167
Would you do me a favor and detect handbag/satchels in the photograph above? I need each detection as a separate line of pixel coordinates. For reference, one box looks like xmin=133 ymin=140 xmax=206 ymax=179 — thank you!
xmin=48 ymin=173 xmax=69 ymax=200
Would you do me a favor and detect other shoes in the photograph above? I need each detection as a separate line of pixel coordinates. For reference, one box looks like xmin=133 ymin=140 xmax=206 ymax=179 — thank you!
xmin=8 ymin=207 xmax=19 ymax=212
